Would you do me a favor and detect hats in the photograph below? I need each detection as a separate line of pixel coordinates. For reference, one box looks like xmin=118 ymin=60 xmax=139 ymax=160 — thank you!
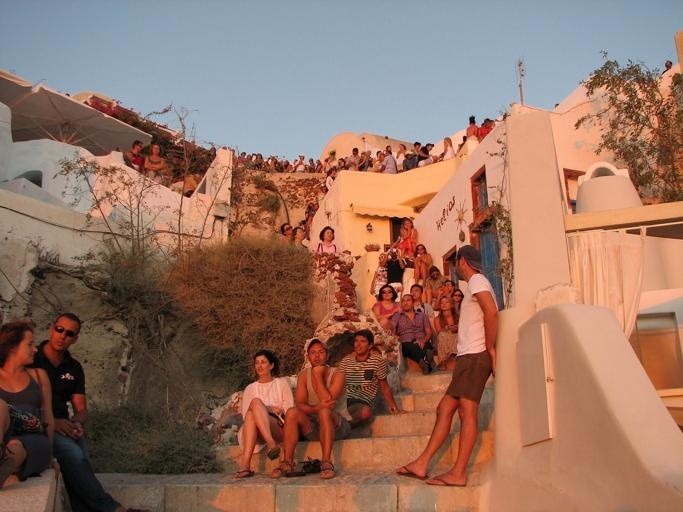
xmin=459 ymin=245 xmax=483 ymax=272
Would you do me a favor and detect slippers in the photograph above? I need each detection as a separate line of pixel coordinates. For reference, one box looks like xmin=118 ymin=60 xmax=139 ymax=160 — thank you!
xmin=267 ymin=446 xmax=280 ymax=460
xmin=234 ymin=469 xmax=255 ymax=478
xmin=426 ymin=475 xmax=466 ymax=487
xmin=396 ymin=465 xmax=428 ymax=480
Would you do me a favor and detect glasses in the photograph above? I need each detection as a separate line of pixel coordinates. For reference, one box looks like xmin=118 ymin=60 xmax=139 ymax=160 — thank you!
xmin=383 ymin=290 xmax=392 ymax=293
xmin=56 ymin=325 xmax=75 ymax=337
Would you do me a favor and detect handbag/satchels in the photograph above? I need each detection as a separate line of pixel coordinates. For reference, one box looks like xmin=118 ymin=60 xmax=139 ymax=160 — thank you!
xmin=6 ymin=404 xmax=45 ymax=436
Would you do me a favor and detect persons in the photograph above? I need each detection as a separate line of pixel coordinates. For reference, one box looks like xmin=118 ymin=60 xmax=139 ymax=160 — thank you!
xmin=229 ymin=147 xmax=339 ymax=172
xmin=1 ymin=320 xmax=61 ymax=489
xmin=341 ymin=118 xmax=496 ymax=174
xmin=281 ymin=167 xmax=338 ymax=253
xmin=397 ymin=245 xmax=498 ymax=488
xmin=125 ymin=140 xmax=198 ymax=198
xmin=662 ymin=61 xmax=672 ymax=74
xmin=233 ymin=329 xmax=407 ymax=481
xmin=371 ymin=217 xmax=465 ymax=376
xmin=26 ymin=313 xmax=152 ymax=511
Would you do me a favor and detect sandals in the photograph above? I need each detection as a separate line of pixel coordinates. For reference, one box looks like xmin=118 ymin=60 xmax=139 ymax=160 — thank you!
xmin=320 ymin=460 xmax=336 ymax=479
xmin=270 ymin=459 xmax=296 ymax=478
